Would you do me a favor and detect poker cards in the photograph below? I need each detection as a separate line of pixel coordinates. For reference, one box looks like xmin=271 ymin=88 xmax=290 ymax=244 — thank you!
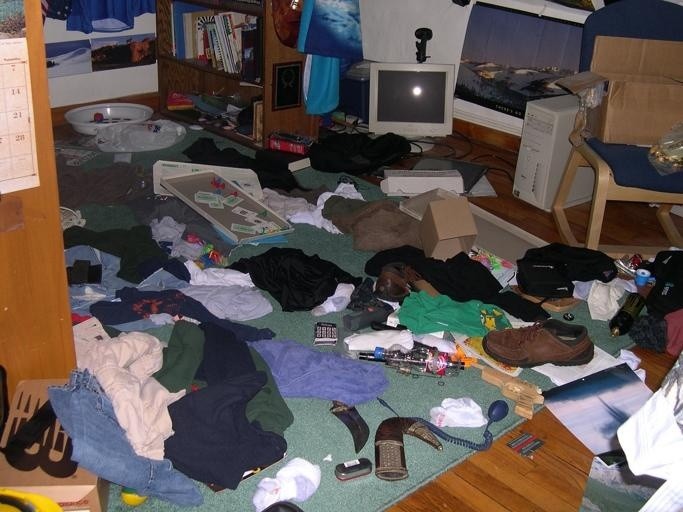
xmin=195 ymin=191 xmax=281 ymax=234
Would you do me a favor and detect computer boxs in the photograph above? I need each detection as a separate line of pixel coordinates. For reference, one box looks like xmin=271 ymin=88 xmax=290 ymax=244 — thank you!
xmin=512 ymin=94 xmax=596 ymax=213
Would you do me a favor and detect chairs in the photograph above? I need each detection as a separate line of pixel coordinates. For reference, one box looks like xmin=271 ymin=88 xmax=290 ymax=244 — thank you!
xmin=551 ymin=0 xmax=683 ymax=254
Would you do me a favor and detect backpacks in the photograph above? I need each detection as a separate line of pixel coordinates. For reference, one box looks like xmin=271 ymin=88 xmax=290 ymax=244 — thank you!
xmin=515 ymin=242 xmax=618 ymax=306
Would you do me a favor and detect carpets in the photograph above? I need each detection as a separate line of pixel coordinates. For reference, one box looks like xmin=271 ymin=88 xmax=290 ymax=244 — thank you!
xmin=52 ymin=104 xmax=640 ymax=512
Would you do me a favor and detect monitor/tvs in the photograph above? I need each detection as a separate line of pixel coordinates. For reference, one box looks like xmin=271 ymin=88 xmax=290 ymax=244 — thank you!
xmin=369 ymin=62 xmax=455 ymax=153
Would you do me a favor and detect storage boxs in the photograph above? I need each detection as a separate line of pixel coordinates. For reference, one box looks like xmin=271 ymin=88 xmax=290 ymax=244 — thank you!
xmin=554 ymin=36 xmax=682 ymax=146
xmin=0 ymin=377 xmax=110 ymax=512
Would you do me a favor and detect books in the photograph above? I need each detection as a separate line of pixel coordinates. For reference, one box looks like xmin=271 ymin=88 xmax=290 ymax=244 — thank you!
xmin=170 ymin=2 xmax=265 ymax=89
xmin=153 ymin=159 xmax=296 ymax=245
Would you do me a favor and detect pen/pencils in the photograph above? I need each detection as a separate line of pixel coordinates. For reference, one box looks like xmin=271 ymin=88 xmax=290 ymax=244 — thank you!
xmin=505 ymin=433 xmax=545 ymax=458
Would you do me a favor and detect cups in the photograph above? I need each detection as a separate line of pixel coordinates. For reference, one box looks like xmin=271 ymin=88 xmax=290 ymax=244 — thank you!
xmin=635 ymin=266 xmax=653 ymax=288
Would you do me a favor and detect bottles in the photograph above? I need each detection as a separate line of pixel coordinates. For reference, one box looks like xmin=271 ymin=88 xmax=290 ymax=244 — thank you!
xmin=609 ymin=292 xmax=646 ymax=338
xmin=373 ymin=344 xmax=461 ymax=376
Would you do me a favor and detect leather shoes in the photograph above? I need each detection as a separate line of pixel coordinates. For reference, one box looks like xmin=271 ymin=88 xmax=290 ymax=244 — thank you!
xmin=373 ymin=262 xmax=421 ymax=302
xmin=481 ymin=318 xmax=594 ymax=367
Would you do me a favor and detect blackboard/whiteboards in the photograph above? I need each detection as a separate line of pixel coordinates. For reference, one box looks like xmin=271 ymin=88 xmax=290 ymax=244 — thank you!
xmin=160 ymin=170 xmax=295 ymax=243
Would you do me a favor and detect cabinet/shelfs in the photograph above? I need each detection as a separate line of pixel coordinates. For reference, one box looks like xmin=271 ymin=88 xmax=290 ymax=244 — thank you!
xmin=155 ymin=0 xmax=327 ymax=150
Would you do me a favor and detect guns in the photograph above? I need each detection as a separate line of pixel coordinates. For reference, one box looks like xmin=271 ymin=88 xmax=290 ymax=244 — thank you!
xmin=473 ymin=361 xmax=544 ymax=419
